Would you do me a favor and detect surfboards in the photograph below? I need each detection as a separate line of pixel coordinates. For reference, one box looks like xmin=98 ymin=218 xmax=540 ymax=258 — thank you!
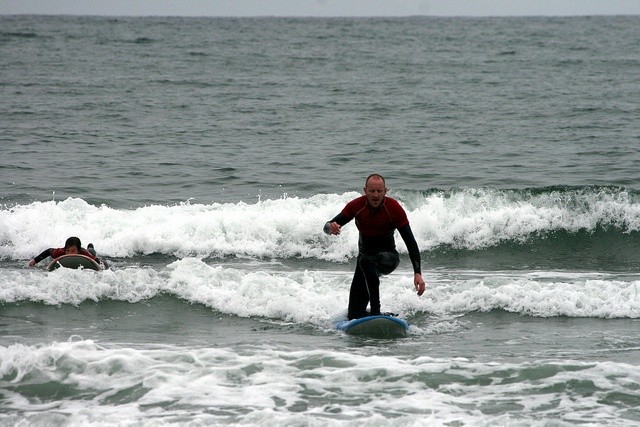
xmin=47 ymin=254 xmax=100 ymax=271
xmin=337 ymin=315 xmax=408 ymax=339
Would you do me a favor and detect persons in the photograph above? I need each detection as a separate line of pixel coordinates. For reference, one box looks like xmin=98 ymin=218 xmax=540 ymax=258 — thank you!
xmin=323 ymin=173 xmax=424 ymax=320
xmin=28 ymin=236 xmax=99 ymax=266
